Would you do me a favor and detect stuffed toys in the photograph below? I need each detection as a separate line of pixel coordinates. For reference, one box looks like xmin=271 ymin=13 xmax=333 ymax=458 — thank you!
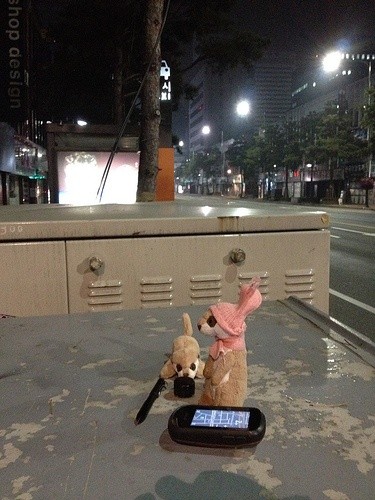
xmin=160 ymin=313 xmax=207 ymax=379
xmin=197 ymin=274 xmax=262 ymax=407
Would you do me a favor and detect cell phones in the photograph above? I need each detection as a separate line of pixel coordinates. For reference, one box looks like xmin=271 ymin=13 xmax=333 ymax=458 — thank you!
xmin=168 ymin=404 xmax=267 ymax=448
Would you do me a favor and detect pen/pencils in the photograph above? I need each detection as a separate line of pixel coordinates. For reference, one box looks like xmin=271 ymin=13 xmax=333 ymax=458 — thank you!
xmin=135 ymin=378 xmax=165 ymax=424
